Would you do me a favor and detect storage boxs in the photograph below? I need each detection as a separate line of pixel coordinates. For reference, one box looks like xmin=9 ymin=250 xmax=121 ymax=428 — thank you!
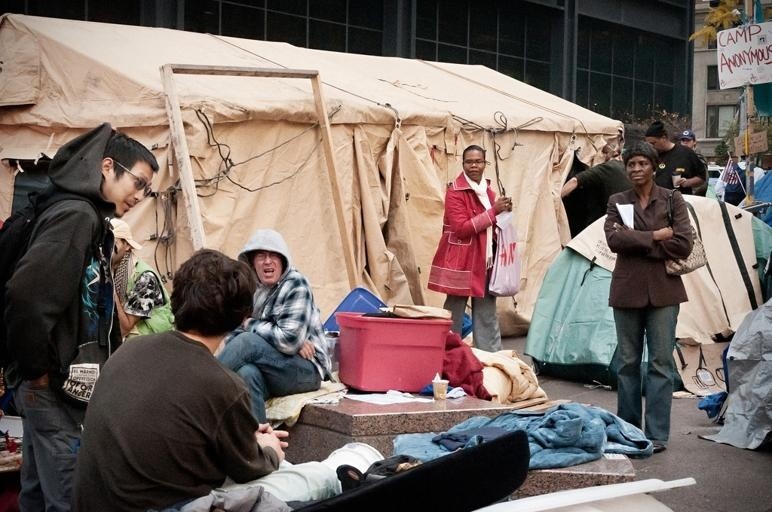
xmin=334 ymin=311 xmax=453 ymax=391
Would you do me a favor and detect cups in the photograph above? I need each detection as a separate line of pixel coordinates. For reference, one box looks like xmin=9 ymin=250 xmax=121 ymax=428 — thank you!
xmin=432 ymin=379 xmax=450 ymax=402
xmin=671 ymin=171 xmax=682 ymax=188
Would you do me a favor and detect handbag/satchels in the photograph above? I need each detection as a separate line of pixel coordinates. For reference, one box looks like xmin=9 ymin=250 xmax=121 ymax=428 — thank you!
xmin=665 ymin=188 xmax=707 ymax=276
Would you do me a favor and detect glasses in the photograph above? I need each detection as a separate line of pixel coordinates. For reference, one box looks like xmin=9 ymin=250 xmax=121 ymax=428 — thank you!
xmin=462 ymin=158 xmax=484 ymax=166
xmin=253 ymin=251 xmax=283 ymax=263
xmin=113 ymin=160 xmax=152 ymax=196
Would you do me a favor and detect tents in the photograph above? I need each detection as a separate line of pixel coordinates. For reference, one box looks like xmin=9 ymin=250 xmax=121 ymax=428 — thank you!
xmin=739 ymin=163 xmax=772 ymax=228
xmin=522 ymin=195 xmax=772 ymax=399
xmin=1 ymin=12 xmax=625 ymax=338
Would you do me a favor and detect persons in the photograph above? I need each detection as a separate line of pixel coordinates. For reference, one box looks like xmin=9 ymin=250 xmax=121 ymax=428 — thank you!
xmin=428 ymin=146 xmax=512 ymax=351
xmin=644 ymin=122 xmax=706 ymax=196
xmin=604 ymin=140 xmax=693 ymax=453
xmin=105 ymin=217 xmax=174 ymax=341
xmin=214 ymin=230 xmax=331 ymax=425
xmin=678 ymin=130 xmax=709 ymax=197
xmin=71 ymin=249 xmax=290 ymax=512
xmin=562 ymin=143 xmax=631 ymax=203
xmin=722 ymin=155 xmax=765 ymax=206
xmin=0 ymin=123 xmax=159 ymax=512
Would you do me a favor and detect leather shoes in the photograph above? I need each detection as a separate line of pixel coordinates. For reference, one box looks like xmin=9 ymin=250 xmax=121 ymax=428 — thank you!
xmin=653 ymin=444 xmax=666 ymax=453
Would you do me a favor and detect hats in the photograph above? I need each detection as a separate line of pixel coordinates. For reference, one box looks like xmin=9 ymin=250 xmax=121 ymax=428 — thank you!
xmin=645 ymin=122 xmax=665 ymax=137
xmin=110 ymin=218 xmax=142 ymax=249
xmin=679 ymin=131 xmax=695 ymax=140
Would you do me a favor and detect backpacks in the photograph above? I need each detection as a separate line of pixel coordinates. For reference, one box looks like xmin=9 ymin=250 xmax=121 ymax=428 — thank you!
xmin=725 ymin=163 xmax=748 ymax=206
xmin=0 ymin=189 xmax=106 ymax=368
